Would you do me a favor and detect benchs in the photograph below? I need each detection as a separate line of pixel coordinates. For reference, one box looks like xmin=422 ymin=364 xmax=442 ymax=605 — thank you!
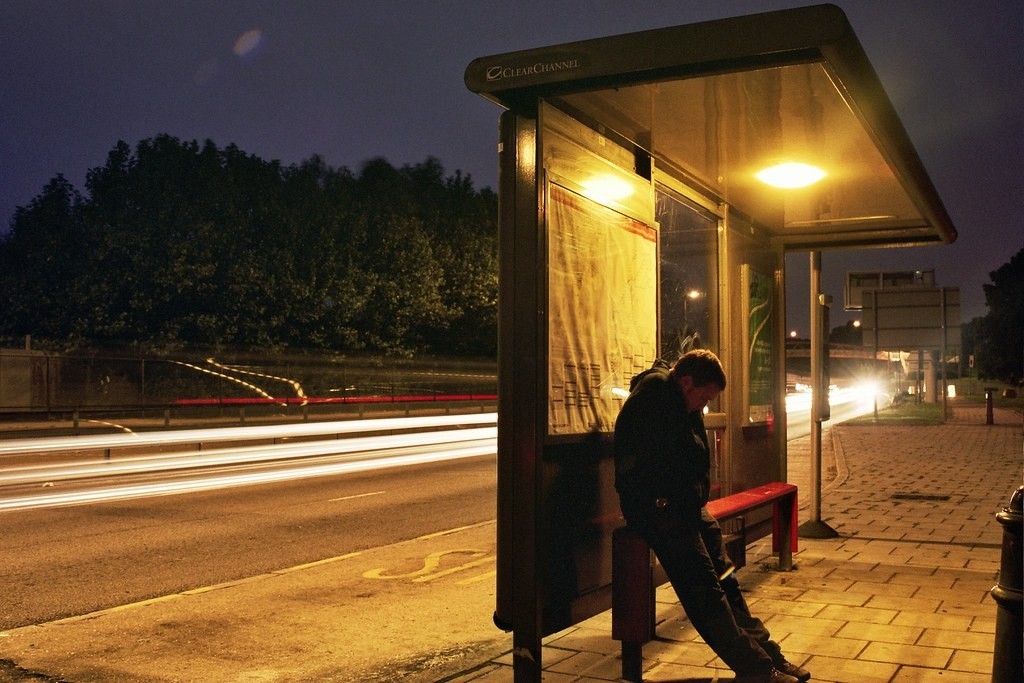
xmin=611 ymin=482 xmax=800 ymax=681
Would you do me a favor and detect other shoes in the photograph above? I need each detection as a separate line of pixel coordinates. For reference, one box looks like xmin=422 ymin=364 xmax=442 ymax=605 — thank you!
xmin=734 ymin=667 xmax=799 ymax=683
xmin=775 ymin=659 xmax=810 ymax=682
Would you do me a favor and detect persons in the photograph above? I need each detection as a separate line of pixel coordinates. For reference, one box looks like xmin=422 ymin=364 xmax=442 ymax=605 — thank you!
xmin=613 ymin=349 xmax=811 ymax=683
